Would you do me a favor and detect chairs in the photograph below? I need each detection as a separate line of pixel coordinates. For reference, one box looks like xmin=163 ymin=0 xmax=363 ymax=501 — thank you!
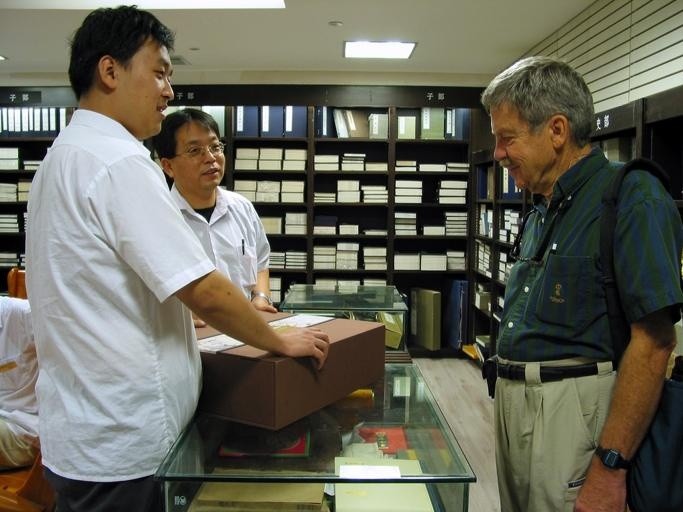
xmin=1 ymin=265 xmax=57 ymax=512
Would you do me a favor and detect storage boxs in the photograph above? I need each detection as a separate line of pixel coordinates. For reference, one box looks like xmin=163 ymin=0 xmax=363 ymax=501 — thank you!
xmin=189 ymin=307 xmax=386 ymax=434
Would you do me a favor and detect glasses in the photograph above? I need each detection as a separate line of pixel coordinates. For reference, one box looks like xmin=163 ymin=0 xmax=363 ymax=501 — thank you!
xmin=174 ymin=143 xmax=225 ymax=160
xmin=509 ymin=207 xmax=561 ymax=267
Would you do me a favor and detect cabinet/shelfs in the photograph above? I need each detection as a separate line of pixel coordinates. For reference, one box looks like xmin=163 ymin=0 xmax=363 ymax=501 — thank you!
xmin=392 ymin=82 xmax=473 ymax=362
xmin=0 ymin=83 xmax=80 ymax=296
xmin=229 ymin=84 xmax=307 ymax=314
xmin=157 ymin=363 xmax=475 ymax=511
xmin=472 ymin=83 xmax=680 ymax=402
xmin=308 ymin=84 xmax=392 ymax=321
xmin=139 ymin=83 xmax=230 ymax=195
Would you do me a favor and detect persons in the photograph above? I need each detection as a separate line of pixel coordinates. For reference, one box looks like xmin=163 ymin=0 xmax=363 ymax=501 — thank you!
xmin=481 ymin=56 xmax=683 ymax=512
xmin=25 ymin=1 xmax=328 ymax=512
xmin=0 ymin=296 xmax=41 ymax=470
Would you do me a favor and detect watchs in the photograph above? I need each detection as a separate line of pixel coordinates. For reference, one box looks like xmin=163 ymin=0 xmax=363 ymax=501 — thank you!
xmin=593 ymin=446 xmax=633 ymax=472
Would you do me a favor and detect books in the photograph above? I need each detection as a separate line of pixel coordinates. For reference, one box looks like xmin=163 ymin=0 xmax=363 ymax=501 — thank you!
xmin=220 ymin=425 xmax=311 ymax=459
xmin=140 ymin=106 xmax=308 ymax=303
xmin=186 ymin=468 xmax=331 ymax=512
xmin=336 ymin=428 xmax=435 ymax=512
xmin=0 ymin=107 xmax=79 ymax=268
xmin=313 ymin=106 xmax=523 ymax=362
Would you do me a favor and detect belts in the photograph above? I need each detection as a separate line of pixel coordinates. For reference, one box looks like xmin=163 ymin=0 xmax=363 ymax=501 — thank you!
xmin=495 ymin=361 xmax=617 ymax=387
